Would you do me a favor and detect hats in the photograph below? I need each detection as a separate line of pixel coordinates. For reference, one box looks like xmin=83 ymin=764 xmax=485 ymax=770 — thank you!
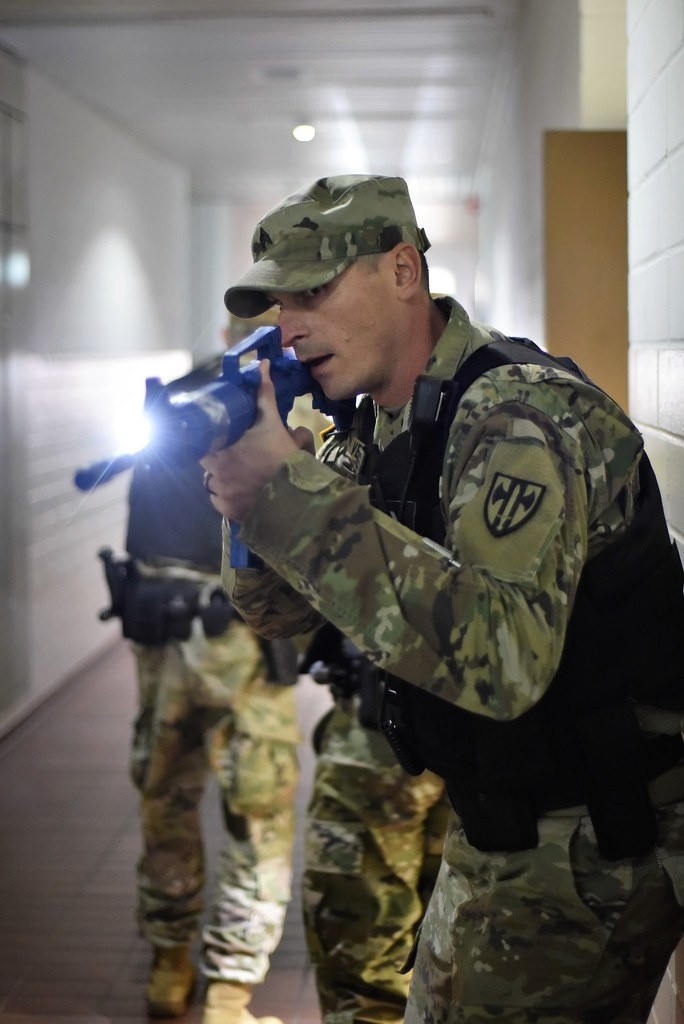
xmin=224 ymin=175 xmax=431 ymax=319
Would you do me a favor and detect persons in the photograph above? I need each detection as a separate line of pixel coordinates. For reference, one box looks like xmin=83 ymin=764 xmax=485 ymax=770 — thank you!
xmin=125 ymin=304 xmax=452 ymax=1024
xmin=202 ymin=176 xmax=684 ymax=1024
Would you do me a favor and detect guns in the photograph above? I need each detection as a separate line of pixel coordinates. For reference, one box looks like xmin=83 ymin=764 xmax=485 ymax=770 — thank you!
xmin=74 ymin=326 xmax=356 ymax=571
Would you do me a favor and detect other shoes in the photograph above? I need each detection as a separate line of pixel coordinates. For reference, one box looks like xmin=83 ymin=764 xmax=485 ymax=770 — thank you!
xmin=202 ymin=981 xmax=283 ymax=1024
xmin=143 ymin=946 xmax=196 ymax=1018
